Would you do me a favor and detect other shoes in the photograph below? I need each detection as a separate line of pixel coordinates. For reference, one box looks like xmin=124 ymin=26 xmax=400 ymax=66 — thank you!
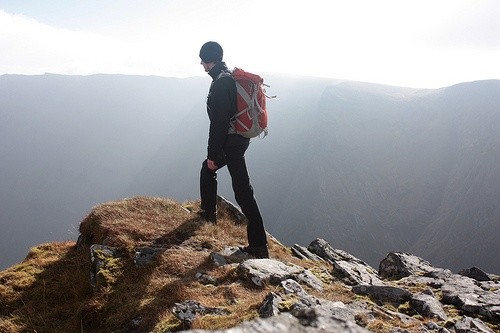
xmin=240 ymin=245 xmax=268 ymax=259
xmin=193 ymin=210 xmax=217 ymax=224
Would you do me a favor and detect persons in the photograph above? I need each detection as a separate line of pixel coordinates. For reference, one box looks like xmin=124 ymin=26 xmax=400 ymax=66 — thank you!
xmin=192 ymin=41 xmax=271 ymax=260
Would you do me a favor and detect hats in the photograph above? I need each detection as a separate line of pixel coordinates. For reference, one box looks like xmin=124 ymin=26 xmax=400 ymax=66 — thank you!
xmin=199 ymin=41 xmax=223 ymax=64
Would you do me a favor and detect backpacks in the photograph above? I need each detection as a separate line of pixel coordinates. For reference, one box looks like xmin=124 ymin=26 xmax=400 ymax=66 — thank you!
xmin=215 ymin=67 xmax=276 ymax=140
